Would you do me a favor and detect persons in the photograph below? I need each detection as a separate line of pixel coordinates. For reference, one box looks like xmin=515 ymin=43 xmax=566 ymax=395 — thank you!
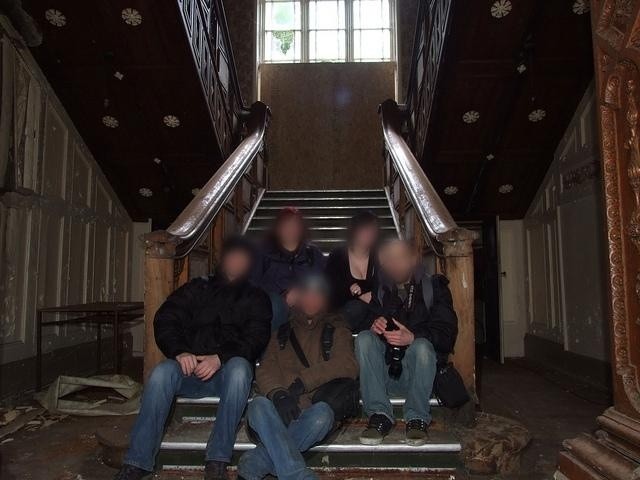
xmin=116 ymin=207 xmax=458 ymax=480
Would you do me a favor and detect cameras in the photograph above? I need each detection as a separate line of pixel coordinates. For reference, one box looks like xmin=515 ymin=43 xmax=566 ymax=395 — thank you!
xmin=377 ymin=314 xmax=409 ymax=378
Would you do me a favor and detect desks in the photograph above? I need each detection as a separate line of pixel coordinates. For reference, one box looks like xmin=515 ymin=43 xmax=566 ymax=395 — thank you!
xmin=35 ymin=301 xmax=143 ymax=390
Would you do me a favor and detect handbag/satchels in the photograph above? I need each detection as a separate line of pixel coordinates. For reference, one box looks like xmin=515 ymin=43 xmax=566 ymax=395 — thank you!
xmin=311 ymin=377 xmax=360 ymax=422
xmin=432 ymin=366 xmax=469 ymax=408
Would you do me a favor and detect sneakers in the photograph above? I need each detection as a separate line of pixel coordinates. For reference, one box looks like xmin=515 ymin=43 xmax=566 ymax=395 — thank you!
xmin=359 ymin=414 xmax=429 ymax=446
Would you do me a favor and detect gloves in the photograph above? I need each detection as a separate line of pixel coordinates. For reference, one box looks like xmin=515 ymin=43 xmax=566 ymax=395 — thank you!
xmin=272 ymin=378 xmax=305 ymax=426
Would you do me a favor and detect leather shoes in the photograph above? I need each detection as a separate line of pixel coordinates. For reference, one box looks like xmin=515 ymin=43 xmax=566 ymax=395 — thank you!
xmin=204 ymin=461 xmax=228 ymax=480
xmin=114 ymin=464 xmax=152 ymax=480
